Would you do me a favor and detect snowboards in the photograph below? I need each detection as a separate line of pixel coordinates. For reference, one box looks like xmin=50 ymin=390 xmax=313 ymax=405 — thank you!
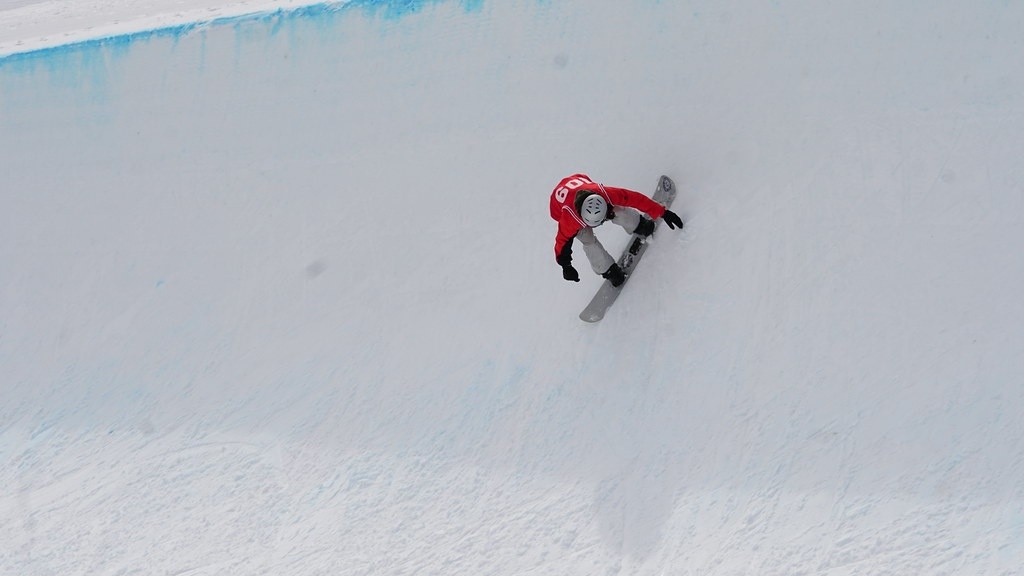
xmin=578 ymin=175 xmax=676 ymax=322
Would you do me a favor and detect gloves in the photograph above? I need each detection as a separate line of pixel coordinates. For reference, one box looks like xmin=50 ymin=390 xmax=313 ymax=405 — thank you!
xmin=562 ymin=265 xmax=580 ymax=282
xmin=661 ymin=210 xmax=683 ymax=230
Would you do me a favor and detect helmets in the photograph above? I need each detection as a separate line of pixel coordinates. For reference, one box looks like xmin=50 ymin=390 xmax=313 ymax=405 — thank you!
xmin=581 ymin=194 xmax=607 ymax=226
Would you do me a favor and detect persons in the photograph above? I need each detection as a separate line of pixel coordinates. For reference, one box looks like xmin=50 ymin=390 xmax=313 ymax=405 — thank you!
xmin=550 ymin=174 xmax=683 ymax=287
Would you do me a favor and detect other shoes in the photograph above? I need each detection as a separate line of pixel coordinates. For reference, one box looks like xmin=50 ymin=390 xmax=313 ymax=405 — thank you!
xmin=633 ymin=215 xmax=654 ymax=237
xmin=602 ymin=263 xmax=627 ymax=287
